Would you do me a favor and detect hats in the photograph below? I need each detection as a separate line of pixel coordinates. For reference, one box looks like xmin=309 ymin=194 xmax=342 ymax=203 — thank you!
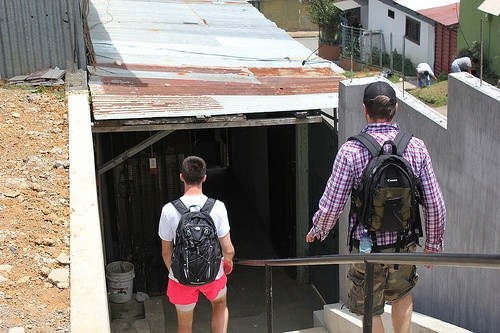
xmin=364 ymin=81 xmax=396 ymax=107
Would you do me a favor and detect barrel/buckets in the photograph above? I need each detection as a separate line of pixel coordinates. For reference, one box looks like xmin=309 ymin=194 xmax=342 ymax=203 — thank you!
xmin=106 ymin=261 xmax=135 ymax=303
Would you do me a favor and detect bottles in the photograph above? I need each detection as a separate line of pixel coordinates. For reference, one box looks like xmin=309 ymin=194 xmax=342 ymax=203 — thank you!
xmin=359 ymin=229 xmax=372 ymax=253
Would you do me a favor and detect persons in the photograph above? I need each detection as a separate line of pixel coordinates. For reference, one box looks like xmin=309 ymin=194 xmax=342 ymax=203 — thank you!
xmin=416 ymin=63 xmax=436 ymax=86
xmin=158 ymin=156 xmax=234 ymax=333
xmin=451 ymin=56 xmax=478 ymax=73
xmin=306 ymin=81 xmax=446 ymax=333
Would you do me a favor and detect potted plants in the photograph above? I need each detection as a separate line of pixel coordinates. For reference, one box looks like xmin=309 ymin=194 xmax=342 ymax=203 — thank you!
xmin=304 ymin=0 xmax=342 ymax=60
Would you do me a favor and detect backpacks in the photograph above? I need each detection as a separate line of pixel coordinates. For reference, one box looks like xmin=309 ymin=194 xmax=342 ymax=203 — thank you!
xmin=346 ymin=133 xmax=415 ymax=231
xmin=170 ymin=198 xmax=222 ymax=286
